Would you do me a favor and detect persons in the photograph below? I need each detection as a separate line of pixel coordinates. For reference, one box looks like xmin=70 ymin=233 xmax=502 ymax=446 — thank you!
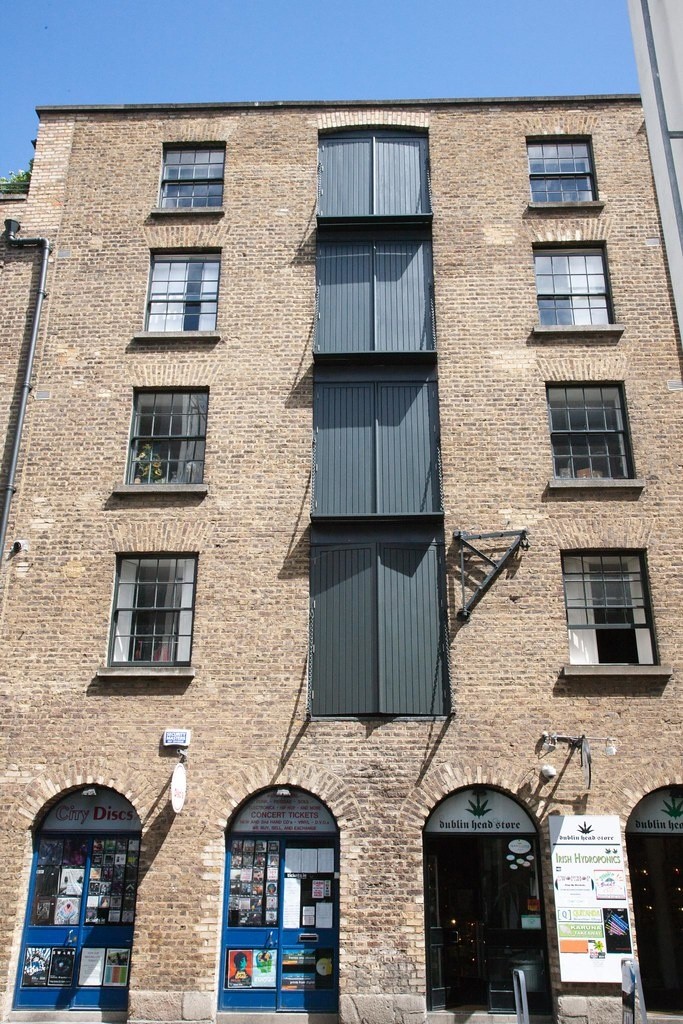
xmin=91 ymin=884 xmax=99 ymax=892
xmin=38 ymin=907 xmax=47 ymax=920
xmin=102 ymin=899 xmax=108 ymax=906
xmin=230 ymin=952 xmax=251 ymax=986
xmin=123 ymin=912 xmax=133 ymax=922
xmin=108 ymin=953 xmax=127 ymax=965
xmin=101 ymin=883 xmax=111 ymax=892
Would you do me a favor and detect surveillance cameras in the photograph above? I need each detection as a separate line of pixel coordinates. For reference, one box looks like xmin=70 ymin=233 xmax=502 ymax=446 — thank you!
xmin=544 ymin=767 xmax=556 ymax=779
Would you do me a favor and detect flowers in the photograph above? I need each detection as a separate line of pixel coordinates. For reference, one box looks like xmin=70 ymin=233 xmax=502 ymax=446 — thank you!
xmin=137 ymin=444 xmax=162 ymax=479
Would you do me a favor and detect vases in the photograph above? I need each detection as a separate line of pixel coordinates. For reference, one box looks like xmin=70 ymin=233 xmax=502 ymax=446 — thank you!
xmin=141 ymin=476 xmax=155 ymax=483
